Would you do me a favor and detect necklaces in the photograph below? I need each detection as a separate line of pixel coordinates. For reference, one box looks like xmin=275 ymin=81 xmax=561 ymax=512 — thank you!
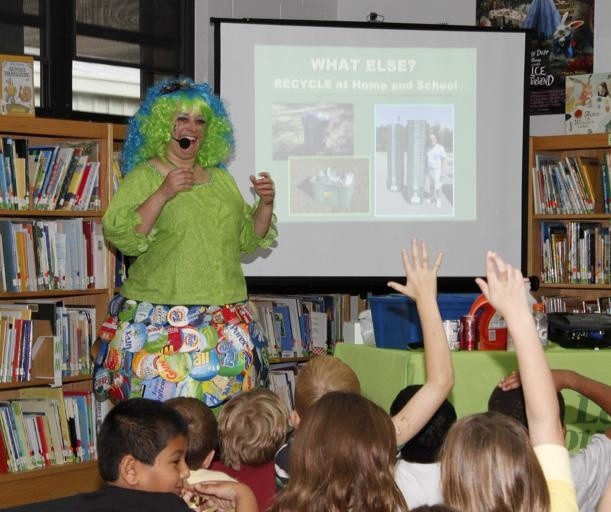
xmin=167 ymin=158 xmax=197 ymax=173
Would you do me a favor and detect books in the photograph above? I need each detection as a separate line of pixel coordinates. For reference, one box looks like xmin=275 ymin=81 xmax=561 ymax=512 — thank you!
xmin=245 ymin=294 xmax=371 ymax=409
xmin=2 ymin=219 xmax=111 ymax=292
xmin=532 ymin=144 xmax=610 ymax=314
xmin=1 ymin=136 xmax=103 ymax=211
xmin=0 ymin=386 xmax=113 ymax=475
xmin=565 ymin=72 xmax=611 ymax=134
xmin=2 ymin=298 xmax=98 ymax=382
xmin=1 ymin=55 xmax=36 ymax=119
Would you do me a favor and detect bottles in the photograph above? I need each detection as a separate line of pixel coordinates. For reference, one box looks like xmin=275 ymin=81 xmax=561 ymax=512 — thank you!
xmin=531 ymin=304 xmax=549 ymax=347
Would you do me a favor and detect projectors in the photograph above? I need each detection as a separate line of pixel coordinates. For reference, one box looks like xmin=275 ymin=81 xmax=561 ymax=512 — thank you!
xmin=546 ymin=312 xmax=611 ymax=350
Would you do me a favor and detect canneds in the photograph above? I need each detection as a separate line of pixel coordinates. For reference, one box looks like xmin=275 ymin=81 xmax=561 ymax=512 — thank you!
xmin=443 ymin=319 xmax=460 ymax=350
xmin=459 ymin=315 xmax=478 ymax=350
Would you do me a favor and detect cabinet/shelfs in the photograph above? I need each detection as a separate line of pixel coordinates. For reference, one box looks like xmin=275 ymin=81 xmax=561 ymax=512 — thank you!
xmin=0 ymin=115 xmax=128 ymax=508
xmin=524 ymin=133 xmax=611 ymax=312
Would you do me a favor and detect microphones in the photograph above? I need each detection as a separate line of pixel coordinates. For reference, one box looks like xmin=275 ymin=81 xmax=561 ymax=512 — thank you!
xmin=171 ymin=134 xmax=191 ymax=149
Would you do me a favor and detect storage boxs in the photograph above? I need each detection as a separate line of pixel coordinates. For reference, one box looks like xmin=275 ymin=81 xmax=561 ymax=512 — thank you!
xmin=369 ymin=296 xmax=479 ymax=350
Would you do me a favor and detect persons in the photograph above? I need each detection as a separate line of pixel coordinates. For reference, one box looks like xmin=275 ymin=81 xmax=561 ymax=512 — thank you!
xmin=426 ymin=133 xmax=448 ymax=207
xmin=262 ymin=240 xmax=610 ymax=510
xmin=55 ymin=390 xmax=292 ymax=511
xmin=95 ymin=77 xmax=280 ymax=419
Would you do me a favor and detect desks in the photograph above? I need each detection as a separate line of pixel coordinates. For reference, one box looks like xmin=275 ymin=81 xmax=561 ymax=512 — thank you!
xmin=333 ymin=341 xmax=610 ymax=457
